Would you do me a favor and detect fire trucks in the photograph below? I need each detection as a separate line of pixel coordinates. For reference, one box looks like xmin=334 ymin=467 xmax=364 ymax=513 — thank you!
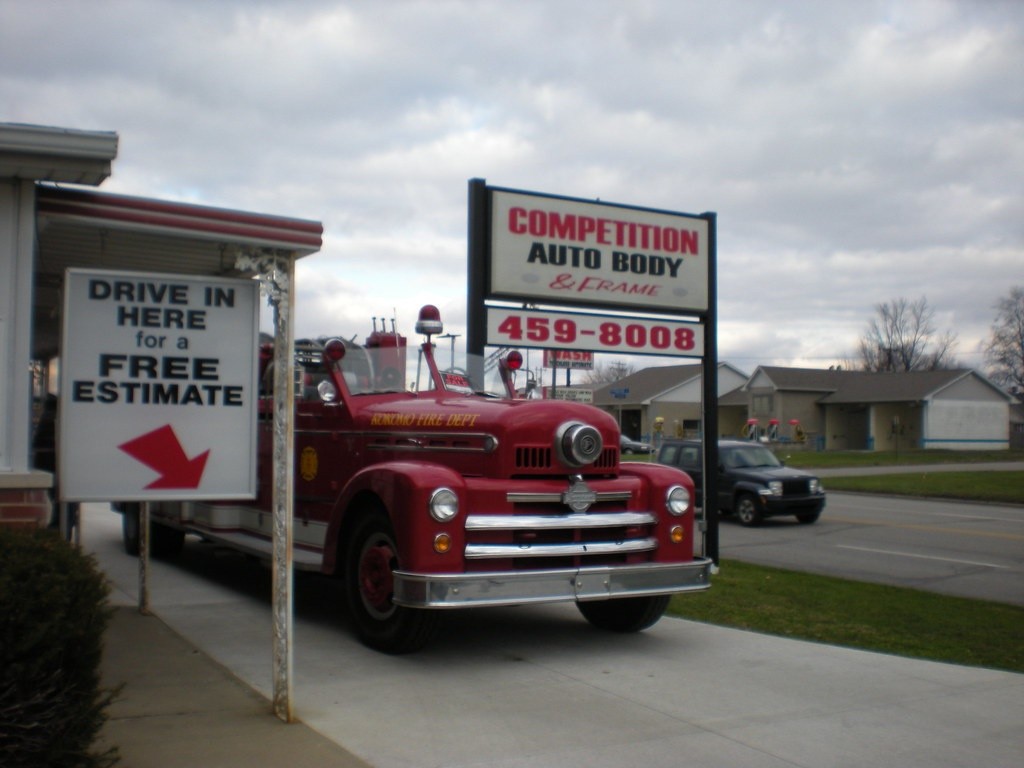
xmin=104 ymin=302 xmax=720 ymax=654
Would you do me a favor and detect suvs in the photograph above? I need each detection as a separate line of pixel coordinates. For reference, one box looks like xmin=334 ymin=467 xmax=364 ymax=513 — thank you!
xmin=654 ymin=438 xmax=826 ymax=527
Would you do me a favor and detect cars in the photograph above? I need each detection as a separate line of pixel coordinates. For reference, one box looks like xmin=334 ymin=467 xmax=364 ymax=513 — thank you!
xmin=620 ymin=435 xmax=656 ymax=455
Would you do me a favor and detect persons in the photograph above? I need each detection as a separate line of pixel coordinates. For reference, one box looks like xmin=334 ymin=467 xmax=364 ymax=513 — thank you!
xmin=33 ymin=392 xmax=77 ymax=541
